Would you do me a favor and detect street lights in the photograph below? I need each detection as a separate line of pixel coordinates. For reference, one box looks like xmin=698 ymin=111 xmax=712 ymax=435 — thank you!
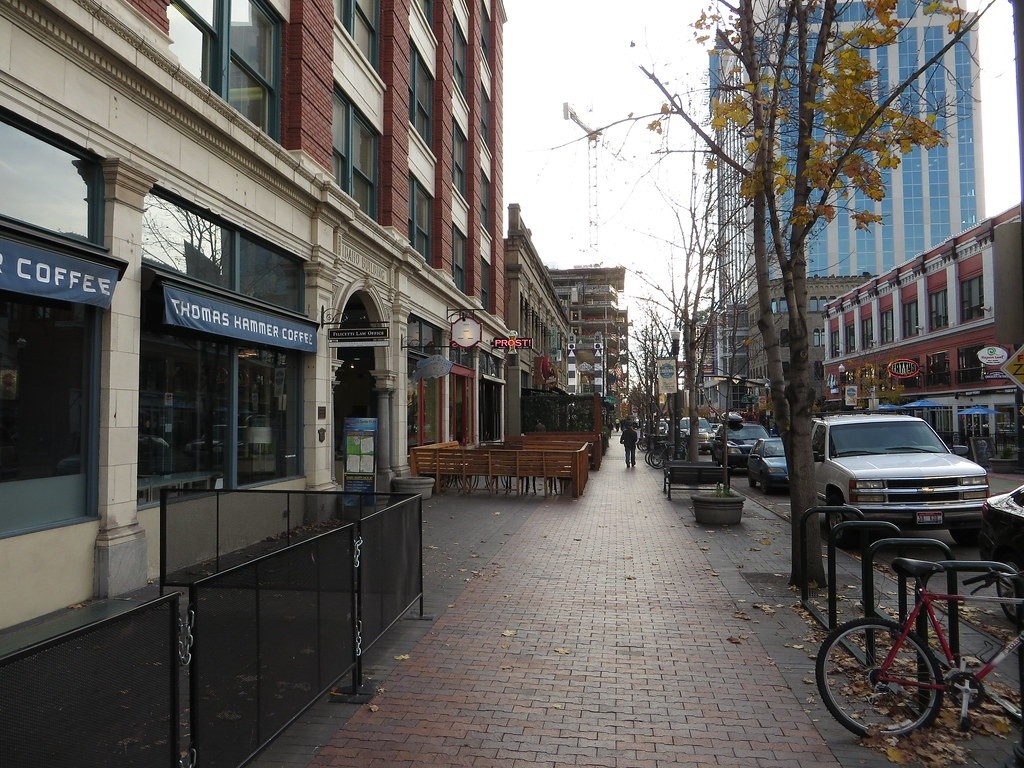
xmin=764 ymin=381 xmax=771 ymax=434
xmin=839 ymin=362 xmax=848 ymax=412
xmin=669 ymin=325 xmax=684 ymax=461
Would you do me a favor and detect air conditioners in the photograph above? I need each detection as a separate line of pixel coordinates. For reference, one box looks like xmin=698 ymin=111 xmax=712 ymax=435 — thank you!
xmin=568 ymin=344 xmax=575 ymax=350
xmin=594 ymin=343 xmax=601 ymax=349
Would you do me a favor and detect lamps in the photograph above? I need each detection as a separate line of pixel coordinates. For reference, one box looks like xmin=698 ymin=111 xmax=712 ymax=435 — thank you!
xmin=242 ymin=427 xmax=275 ymax=462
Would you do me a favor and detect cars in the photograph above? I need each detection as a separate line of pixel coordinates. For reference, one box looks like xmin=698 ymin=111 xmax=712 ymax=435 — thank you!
xmin=633 ymin=414 xmax=721 ymax=470
xmin=976 ymin=484 xmax=1024 ymax=625
xmin=747 ymin=437 xmax=791 ymax=496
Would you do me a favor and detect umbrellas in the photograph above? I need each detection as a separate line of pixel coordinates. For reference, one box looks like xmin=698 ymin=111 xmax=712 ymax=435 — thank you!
xmin=865 ymin=403 xmax=905 ymax=412
xmin=902 ymin=399 xmax=944 ymax=420
xmin=957 ymin=405 xmax=1002 ymax=435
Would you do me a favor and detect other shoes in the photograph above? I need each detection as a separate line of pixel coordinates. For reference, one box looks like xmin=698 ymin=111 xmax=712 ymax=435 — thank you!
xmin=632 ymin=462 xmax=636 ymax=467
xmin=627 ymin=464 xmax=630 ymax=467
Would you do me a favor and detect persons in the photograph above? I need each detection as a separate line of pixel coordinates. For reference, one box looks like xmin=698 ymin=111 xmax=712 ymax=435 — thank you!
xmin=615 ymin=417 xmax=620 ymax=431
xmin=620 ymin=425 xmax=637 ymax=468
xmin=535 ymin=419 xmax=545 ymax=433
xmin=620 ymin=418 xmax=632 ymax=431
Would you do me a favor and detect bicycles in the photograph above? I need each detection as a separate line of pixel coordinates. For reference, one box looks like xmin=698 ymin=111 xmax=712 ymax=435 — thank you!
xmin=812 ymin=556 xmax=1024 ymax=740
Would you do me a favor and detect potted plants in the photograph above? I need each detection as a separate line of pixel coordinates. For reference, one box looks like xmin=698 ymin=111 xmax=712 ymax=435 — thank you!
xmin=690 ymin=482 xmax=746 ymax=525
xmin=989 ymin=448 xmax=1017 ymax=473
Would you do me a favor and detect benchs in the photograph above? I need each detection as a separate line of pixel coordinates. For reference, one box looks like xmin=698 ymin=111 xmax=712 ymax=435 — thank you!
xmin=665 ymin=466 xmax=732 ymax=501
xmin=410 ymin=431 xmax=593 ymax=499
xmin=662 ymin=459 xmax=718 ymax=495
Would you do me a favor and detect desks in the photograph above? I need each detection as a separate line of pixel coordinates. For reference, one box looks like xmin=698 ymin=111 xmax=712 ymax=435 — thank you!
xmin=138 ymin=471 xmax=222 ymax=504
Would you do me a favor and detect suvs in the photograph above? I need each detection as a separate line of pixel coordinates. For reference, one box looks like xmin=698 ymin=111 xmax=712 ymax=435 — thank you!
xmin=710 ymin=411 xmax=776 ymax=469
xmin=810 ymin=410 xmax=991 ymax=550
